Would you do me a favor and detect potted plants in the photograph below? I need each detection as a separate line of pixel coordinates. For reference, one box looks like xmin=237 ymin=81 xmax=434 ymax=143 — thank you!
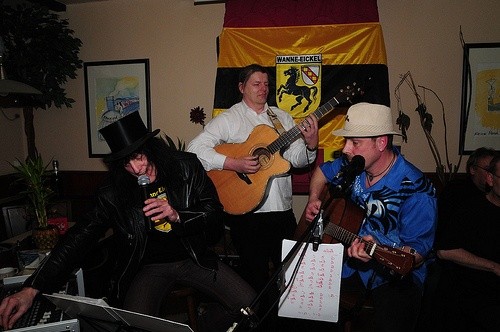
xmin=0 ymin=0 xmax=85 ymax=109
xmin=5 ymin=149 xmax=58 ymax=250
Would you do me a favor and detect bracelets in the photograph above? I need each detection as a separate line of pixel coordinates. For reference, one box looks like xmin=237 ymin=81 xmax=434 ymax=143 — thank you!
xmin=173 ymin=210 xmax=181 ymax=223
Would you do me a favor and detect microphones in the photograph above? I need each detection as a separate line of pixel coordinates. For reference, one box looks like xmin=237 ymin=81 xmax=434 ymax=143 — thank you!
xmin=336 ymin=155 xmax=366 ymax=192
xmin=138 ymin=175 xmax=154 ymax=232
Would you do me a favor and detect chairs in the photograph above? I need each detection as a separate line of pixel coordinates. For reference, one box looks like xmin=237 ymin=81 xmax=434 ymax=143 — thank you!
xmin=2 ymin=201 xmax=72 ymax=238
xmin=423 ymin=256 xmax=500 ymax=332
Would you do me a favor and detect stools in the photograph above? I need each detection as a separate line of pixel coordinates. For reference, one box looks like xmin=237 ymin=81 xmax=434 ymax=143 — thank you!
xmin=338 ymin=292 xmax=374 ymax=332
xmin=167 ymin=285 xmax=200 ymax=332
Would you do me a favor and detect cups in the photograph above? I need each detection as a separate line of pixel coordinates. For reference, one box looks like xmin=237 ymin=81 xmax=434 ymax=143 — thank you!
xmin=0 ymin=268 xmax=18 ymax=280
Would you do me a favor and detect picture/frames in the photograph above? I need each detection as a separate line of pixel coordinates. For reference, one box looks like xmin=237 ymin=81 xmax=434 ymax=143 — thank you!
xmin=83 ymin=59 xmax=151 ymax=158
xmin=458 ymin=42 xmax=500 ymax=156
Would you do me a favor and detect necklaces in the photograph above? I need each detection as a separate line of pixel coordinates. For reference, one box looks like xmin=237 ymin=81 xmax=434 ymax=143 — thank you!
xmin=364 ymin=150 xmax=396 ymax=187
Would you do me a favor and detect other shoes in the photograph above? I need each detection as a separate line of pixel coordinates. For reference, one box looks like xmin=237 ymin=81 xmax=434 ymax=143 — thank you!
xmin=183 ymin=302 xmax=208 ymax=324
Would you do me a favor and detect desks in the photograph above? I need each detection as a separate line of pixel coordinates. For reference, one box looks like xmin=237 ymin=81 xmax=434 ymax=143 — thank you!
xmin=0 ymin=221 xmax=76 ymax=275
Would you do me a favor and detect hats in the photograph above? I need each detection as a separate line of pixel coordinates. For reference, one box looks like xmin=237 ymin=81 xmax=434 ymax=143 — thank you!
xmin=98 ymin=110 xmax=161 ymax=162
xmin=332 ymin=102 xmax=405 ymax=138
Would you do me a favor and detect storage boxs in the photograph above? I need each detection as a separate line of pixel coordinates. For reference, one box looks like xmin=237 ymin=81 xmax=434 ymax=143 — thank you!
xmin=47 ymin=216 xmax=67 ymax=236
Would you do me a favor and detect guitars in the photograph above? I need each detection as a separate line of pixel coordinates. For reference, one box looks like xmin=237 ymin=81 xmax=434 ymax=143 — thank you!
xmin=205 ymin=81 xmax=368 ymax=214
xmin=294 ymin=183 xmax=417 ymax=280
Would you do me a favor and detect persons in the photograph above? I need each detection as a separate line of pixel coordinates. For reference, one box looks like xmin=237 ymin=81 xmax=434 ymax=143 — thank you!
xmin=0 ymin=110 xmax=260 ymax=332
xmin=305 ymin=101 xmax=438 ymax=332
xmin=185 ymin=64 xmax=318 ymax=284
xmin=436 ymin=147 xmax=498 ymax=235
xmin=432 ymin=152 xmax=500 ymax=276
